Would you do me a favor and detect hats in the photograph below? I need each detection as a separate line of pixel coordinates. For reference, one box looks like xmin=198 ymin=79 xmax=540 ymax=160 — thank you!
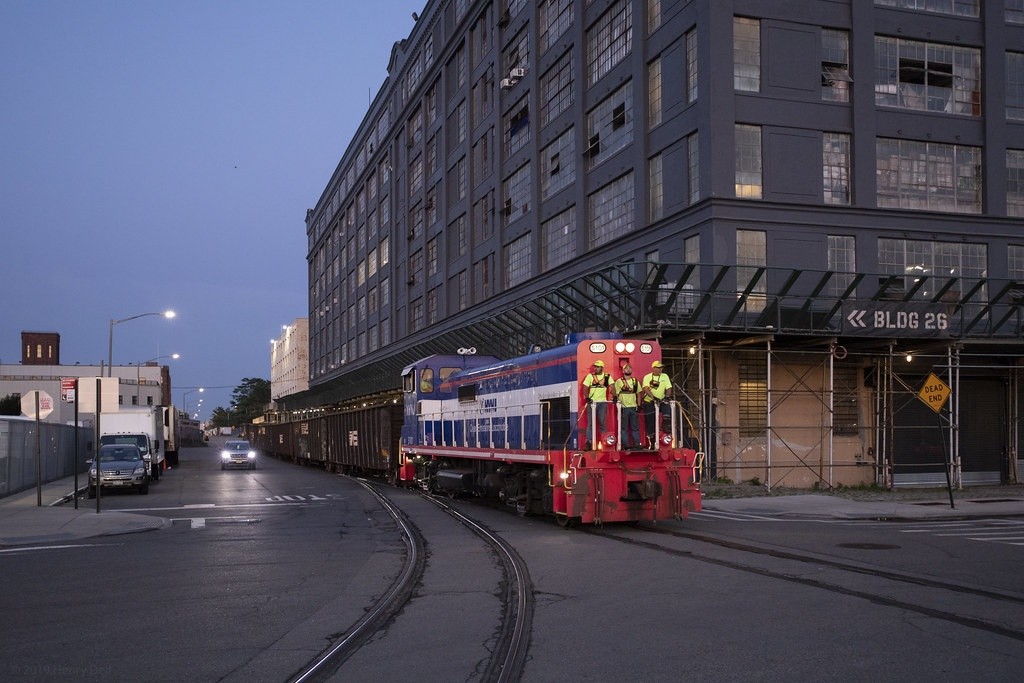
xmin=593 ymin=360 xmax=604 ymax=368
xmin=623 ymin=364 xmax=631 ymax=370
xmin=652 ymin=361 xmax=665 ymax=368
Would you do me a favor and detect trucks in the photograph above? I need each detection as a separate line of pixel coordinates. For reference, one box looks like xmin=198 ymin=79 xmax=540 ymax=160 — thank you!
xmin=94 ymin=406 xmax=166 ymax=485
xmin=162 ymin=406 xmax=182 ymax=468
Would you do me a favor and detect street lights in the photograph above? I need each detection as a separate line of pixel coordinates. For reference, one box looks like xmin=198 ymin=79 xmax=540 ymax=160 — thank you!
xmin=183 ymin=388 xmax=204 ymax=412
xmin=136 ymin=354 xmax=179 ymax=405
xmin=107 ymin=311 xmax=176 ymax=378
xmin=186 ymin=399 xmax=203 ymax=419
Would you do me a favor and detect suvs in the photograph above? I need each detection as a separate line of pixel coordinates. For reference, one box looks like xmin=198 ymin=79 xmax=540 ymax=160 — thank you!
xmin=218 ymin=440 xmax=257 ymax=470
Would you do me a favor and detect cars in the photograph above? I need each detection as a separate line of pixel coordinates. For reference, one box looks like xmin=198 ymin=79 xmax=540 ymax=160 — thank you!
xmin=84 ymin=442 xmax=151 ymax=498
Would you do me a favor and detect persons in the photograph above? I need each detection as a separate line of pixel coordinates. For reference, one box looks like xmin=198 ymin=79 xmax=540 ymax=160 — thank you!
xmin=582 ymin=360 xmax=617 ymax=450
xmin=642 ymin=361 xmax=673 ymax=450
xmin=615 ymin=363 xmax=645 ymax=451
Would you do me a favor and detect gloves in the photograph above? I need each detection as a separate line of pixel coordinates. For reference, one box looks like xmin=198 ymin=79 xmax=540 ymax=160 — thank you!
xmin=663 ymin=397 xmax=670 ymax=404
xmin=652 ymin=396 xmax=661 ymax=404
xmin=612 ymin=396 xmax=617 ymax=403
xmin=586 ymin=397 xmax=593 ymax=404
xmin=636 ymin=406 xmax=642 ymax=413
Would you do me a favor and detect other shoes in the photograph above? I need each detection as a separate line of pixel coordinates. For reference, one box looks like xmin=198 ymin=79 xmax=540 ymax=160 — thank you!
xmin=623 ymin=443 xmax=630 ymax=450
xmin=633 ymin=442 xmax=645 ymax=450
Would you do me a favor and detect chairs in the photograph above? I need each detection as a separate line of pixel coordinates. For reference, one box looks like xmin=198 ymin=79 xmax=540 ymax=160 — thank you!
xmin=101 ymin=450 xmax=114 ymax=461
xmin=123 ymin=452 xmax=136 ymax=459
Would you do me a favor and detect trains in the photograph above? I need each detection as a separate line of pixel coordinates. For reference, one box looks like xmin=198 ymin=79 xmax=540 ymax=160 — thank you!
xmin=242 ymin=331 xmax=706 ymax=532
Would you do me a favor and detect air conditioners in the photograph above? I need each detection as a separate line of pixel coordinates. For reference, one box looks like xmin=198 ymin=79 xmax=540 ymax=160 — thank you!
xmin=500 ymin=69 xmax=524 ymax=89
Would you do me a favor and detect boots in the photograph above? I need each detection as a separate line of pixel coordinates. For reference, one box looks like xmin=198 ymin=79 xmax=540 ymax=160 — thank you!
xmin=650 ymin=438 xmax=655 ymax=450
xmin=584 ymin=441 xmax=591 ymax=451
xmin=660 ymin=420 xmax=671 ymax=434
